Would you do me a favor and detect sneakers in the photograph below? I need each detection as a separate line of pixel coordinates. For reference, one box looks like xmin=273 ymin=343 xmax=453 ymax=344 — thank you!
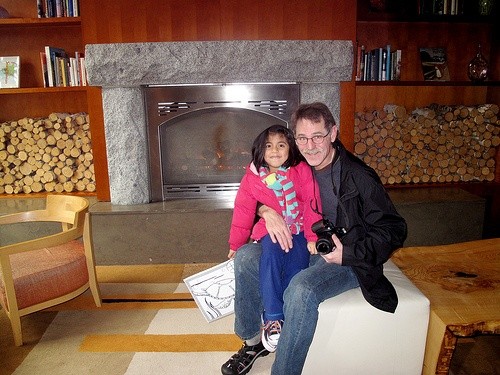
xmin=261 ymin=313 xmax=282 ymax=352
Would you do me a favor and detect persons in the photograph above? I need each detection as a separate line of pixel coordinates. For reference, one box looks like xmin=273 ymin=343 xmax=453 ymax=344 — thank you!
xmin=228 ymin=125 xmax=323 ymax=353
xmin=221 ymin=102 xmax=408 ymax=375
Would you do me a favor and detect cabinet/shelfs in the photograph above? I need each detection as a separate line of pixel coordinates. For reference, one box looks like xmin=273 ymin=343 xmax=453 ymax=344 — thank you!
xmin=0 ymin=0 xmax=97 ymax=213
xmin=355 ymin=11 xmax=500 ymax=204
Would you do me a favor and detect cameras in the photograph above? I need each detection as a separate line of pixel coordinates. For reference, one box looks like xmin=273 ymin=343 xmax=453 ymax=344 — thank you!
xmin=312 ymin=218 xmax=347 ymax=254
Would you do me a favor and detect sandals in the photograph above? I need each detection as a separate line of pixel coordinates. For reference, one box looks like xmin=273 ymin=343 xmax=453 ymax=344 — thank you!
xmin=221 ymin=341 xmax=270 ymax=375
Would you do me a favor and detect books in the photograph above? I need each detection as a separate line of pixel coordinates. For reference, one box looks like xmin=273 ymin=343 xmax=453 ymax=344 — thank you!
xmin=40 ymin=46 xmax=86 ymax=89
xmin=37 ymin=0 xmax=78 ymax=18
xmin=355 ymin=45 xmax=401 ymax=81
xmin=420 ymin=48 xmax=450 ymax=81
xmin=416 ymin=0 xmax=464 ymax=17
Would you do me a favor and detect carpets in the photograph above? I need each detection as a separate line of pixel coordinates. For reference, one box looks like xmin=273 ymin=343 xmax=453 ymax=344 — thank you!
xmin=0 ymin=264 xmax=275 ymax=375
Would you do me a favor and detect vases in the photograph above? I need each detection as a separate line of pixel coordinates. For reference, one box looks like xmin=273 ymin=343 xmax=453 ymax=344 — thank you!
xmin=468 ymin=44 xmax=489 ymax=81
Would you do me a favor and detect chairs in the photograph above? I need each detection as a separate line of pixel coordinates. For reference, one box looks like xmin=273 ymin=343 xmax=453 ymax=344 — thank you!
xmin=0 ymin=193 xmax=103 ymax=348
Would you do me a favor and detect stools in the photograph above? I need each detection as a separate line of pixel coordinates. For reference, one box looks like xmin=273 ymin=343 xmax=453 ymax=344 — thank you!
xmin=300 ymin=258 xmax=432 ymax=375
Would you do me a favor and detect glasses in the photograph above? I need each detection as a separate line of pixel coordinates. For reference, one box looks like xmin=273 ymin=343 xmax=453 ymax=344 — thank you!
xmin=293 ymin=129 xmax=330 ymax=145
